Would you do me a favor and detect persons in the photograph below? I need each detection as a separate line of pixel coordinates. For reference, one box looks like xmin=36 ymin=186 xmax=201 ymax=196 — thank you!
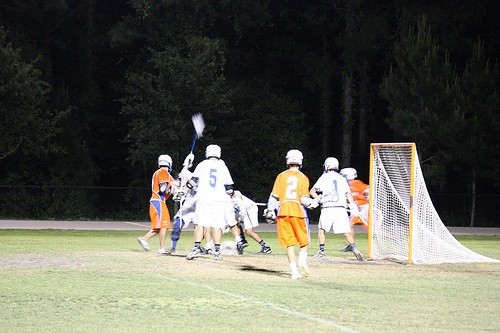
xmin=138 ymin=154 xmax=178 ymax=255
xmin=264 ymin=150 xmax=324 ymax=281
xmin=309 ymin=156 xmax=363 ymax=261
xmin=339 ymin=167 xmax=406 ymax=253
xmin=160 ymin=145 xmax=272 ymax=260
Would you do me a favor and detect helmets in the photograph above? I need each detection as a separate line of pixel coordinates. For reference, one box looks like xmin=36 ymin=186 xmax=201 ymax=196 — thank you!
xmin=157 ymin=154 xmax=173 ymax=172
xmin=323 ymin=157 xmax=339 ymax=171
xmin=205 ymin=145 xmax=222 ymax=159
xmin=340 ymin=168 xmax=358 ymax=180
xmin=285 ymin=150 xmax=304 ymax=165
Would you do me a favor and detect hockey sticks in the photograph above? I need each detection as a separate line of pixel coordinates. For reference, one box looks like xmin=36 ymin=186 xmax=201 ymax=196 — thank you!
xmin=254 ymin=202 xmax=268 ymax=206
xmin=346 ymin=207 xmax=382 ymax=229
xmin=188 ymin=113 xmax=205 ymax=167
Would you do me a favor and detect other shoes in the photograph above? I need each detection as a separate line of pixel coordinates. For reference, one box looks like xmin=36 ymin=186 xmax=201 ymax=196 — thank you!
xmin=312 ymin=251 xmax=324 ymax=259
xmin=236 ymin=240 xmax=248 ymax=255
xmin=186 ymin=246 xmax=203 ymax=260
xmin=170 ymin=248 xmax=176 ymax=253
xmin=256 ymin=246 xmax=272 ymax=254
xmin=205 ymin=249 xmax=213 ymax=255
xmin=291 ymin=272 xmax=302 ymax=280
xmin=158 ymin=247 xmax=171 ymax=254
xmin=352 ymin=248 xmax=364 ymax=262
xmin=214 ymin=252 xmax=223 ymax=260
xmin=137 ymin=237 xmax=150 ymax=251
xmin=296 ymin=261 xmax=311 ymax=278
